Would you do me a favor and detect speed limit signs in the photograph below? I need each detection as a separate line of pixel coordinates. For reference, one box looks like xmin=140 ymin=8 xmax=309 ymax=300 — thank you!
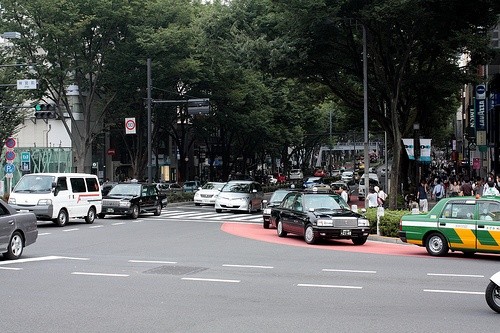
xmin=125 ymin=117 xmax=137 ymax=134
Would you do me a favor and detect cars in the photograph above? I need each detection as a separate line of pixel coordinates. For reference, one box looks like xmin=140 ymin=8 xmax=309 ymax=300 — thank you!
xmin=152 ymin=180 xmax=203 ymax=194
xmin=193 ymin=182 xmax=228 ymax=206
xmin=99 ymin=182 xmax=168 ymax=220
xmin=265 ymin=153 xmax=389 ymax=203
xmin=260 ymin=190 xmax=295 ymax=229
xmin=214 ymin=181 xmax=264 ymax=213
xmin=269 ymin=188 xmax=373 ymax=244
xmin=398 ymin=195 xmax=500 ymax=257
xmin=0 ymin=199 xmax=38 ymax=260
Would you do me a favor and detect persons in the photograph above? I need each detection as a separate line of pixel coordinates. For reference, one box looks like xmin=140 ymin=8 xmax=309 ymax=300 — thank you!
xmin=100 ymin=169 xmax=252 ymax=191
xmin=340 ymin=156 xmax=500 ymax=214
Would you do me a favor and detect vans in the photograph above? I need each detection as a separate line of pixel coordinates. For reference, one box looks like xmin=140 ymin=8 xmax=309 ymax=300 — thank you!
xmin=7 ymin=172 xmax=102 ymax=228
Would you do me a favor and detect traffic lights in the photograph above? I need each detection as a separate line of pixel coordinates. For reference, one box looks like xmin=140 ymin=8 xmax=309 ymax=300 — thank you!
xmin=34 ymin=104 xmax=55 ymax=111
xmin=33 ymin=111 xmax=55 ymax=120
